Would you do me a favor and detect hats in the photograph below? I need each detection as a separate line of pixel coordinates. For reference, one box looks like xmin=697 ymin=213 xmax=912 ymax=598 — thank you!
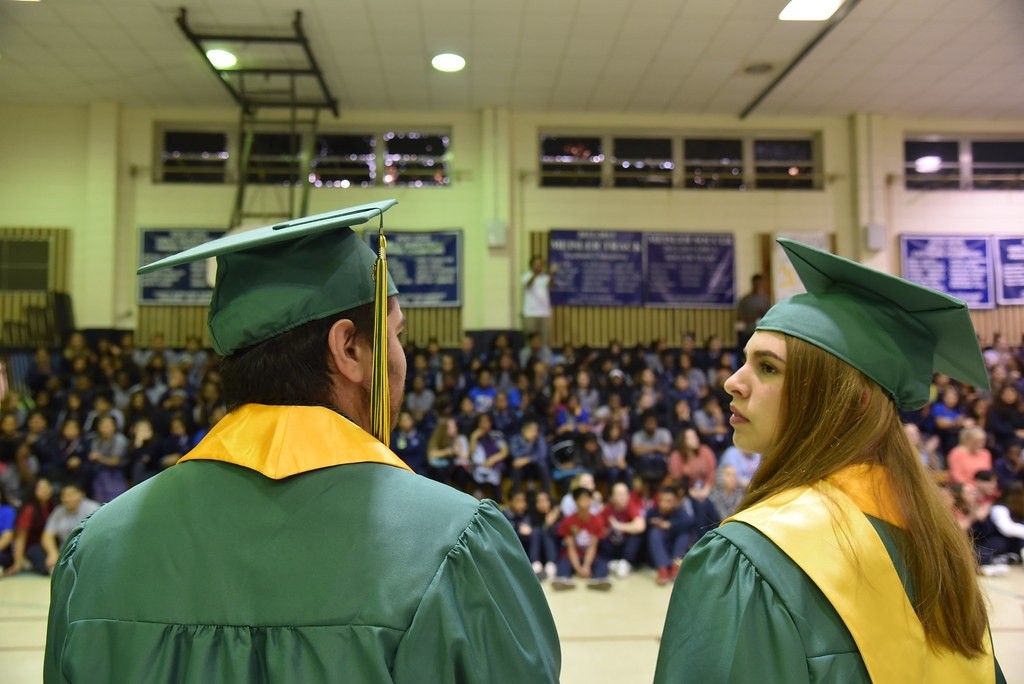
xmin=609 ymin=369 xmax=623 ymax=378
xmin=136 ymin=198 xmax=398 ymax=451
xmin=756 ymin=237 xmax=992 ymax=413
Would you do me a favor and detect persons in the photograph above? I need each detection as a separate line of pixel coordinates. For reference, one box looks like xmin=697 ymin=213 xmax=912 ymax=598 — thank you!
xmin=735 ymin=275 xmax=771 ymax=357
xmin=0 ymin=332 xmax=226 ymax=573
xmin=519 ymin=255 xmax=555 ymax=345
xmin=652 ymin=237 xmax=1005 ymax=684
xmin=899 ymin=332 xmax=1024 ymax=574
xmin=392 ymin=331 xmax=758 ymax=591
xmin=43 ymin=200 xmax=565 ymax=684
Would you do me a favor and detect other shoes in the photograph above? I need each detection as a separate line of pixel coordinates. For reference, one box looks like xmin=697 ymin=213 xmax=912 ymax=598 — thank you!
xmin=529 ymin=559 xmax=685 ymax=586
